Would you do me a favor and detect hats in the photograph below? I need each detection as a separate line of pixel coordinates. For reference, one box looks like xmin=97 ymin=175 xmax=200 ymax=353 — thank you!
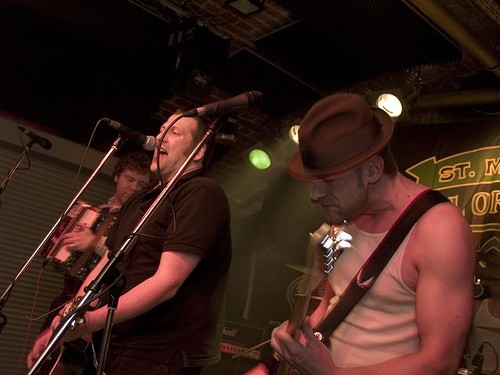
xmin=286 ymin=92 xmax=396 ymax=184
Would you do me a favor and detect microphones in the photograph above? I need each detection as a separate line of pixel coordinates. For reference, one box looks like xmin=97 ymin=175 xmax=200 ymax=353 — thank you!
xmin=104 ymin=118 xmax=156 ymax=151
xmin=18 ymin=126 xmax=52 ymax=150
xmin=187 ymin=91 xmax=263 ymax=117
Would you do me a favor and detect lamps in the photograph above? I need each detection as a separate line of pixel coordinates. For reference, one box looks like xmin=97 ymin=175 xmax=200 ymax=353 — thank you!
xmin=367 ymin=69 xmax=424 ymax=122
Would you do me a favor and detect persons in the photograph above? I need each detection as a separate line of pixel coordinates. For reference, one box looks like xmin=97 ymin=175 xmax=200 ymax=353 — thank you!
xmin=25 ymin=108 xmax=234 ymax=374
xmin=245 ymin=92 xmax=478 ymax=375
xmin=41 ymin=150 xmax=158 ymax=333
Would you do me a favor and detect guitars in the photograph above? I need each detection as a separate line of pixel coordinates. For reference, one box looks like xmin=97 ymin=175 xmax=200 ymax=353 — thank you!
xmin=33 ymin=296 xmax=90 ymax=375
xmin=275 ymin=223 xmax=352 ymax=375
